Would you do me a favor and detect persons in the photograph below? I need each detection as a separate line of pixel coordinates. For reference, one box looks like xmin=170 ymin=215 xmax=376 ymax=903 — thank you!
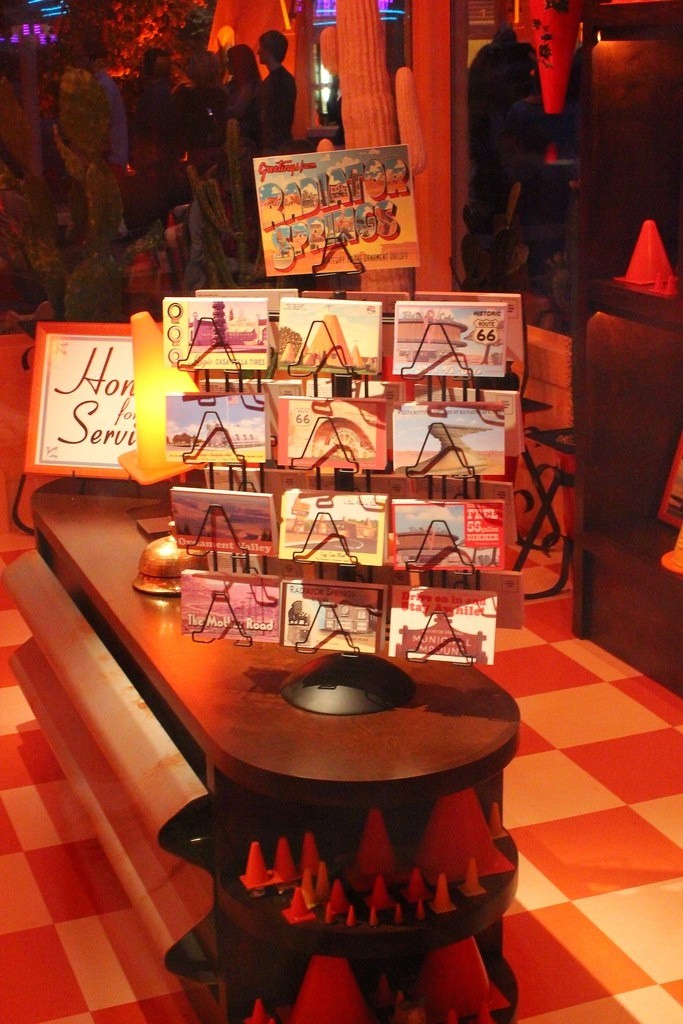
xmin=462 ymin=43 xmax=581 ymax=315
xmin=48 ymin=32 xmax=297 ymax=274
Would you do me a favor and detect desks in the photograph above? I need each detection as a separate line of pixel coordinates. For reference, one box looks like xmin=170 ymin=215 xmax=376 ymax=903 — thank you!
xmin=6 ymin=473 xmax=523 ymax=1024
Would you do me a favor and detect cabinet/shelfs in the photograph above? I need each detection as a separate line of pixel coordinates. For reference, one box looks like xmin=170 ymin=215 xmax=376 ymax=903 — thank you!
xmin=561 ymin=0 xmax=683 ymax=695
